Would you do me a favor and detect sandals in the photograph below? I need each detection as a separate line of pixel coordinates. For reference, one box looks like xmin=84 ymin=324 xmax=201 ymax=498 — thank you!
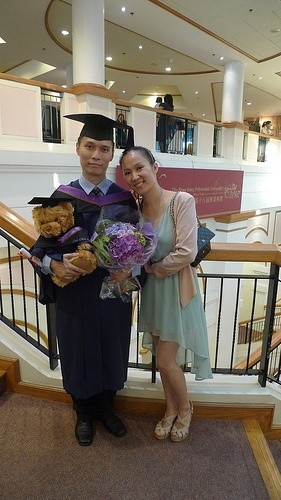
xmin=153 ymin=400 xmax=194 ymax=442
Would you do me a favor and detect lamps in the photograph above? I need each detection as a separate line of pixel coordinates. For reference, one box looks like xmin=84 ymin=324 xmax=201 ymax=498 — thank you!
xmin=165 ymin=58 xmax=174 ymax=72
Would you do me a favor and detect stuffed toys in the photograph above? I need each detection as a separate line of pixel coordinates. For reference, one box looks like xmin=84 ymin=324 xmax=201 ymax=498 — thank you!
xmin=25 ymin=195 xmax=97 ymax=288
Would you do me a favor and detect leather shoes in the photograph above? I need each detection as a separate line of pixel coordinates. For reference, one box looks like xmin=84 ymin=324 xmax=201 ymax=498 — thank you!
xmin=95 ymin=404 xmax=127 ymax=439
xmin=74 ymin=413 xmax=96 ymax=446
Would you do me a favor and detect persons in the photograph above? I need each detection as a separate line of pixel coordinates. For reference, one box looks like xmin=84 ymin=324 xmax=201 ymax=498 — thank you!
xmin=117 ymin=114 xmax=128 ymax=147
xmin=257 ymin=120 xmax=275 ymax=161
xmin=26 ymin=113 xmax=146 ymax=447
xmin=153 ymin=93 xmax=185 ymax=153
xmin=106 ymin=146 xmax=215 ymax=443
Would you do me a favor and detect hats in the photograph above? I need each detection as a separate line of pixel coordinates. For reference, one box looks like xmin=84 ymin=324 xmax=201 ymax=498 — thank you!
xmin=63 ymin=114 xmax=134 ymax=153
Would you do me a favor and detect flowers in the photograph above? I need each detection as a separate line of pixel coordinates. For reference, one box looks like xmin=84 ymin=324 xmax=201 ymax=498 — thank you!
xmin=90 ymin=222 xmax=161 ymax=299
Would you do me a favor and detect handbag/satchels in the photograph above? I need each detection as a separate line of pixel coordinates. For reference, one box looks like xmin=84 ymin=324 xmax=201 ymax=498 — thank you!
xmin=169 ymin=191 xmax=216 ymax=268
